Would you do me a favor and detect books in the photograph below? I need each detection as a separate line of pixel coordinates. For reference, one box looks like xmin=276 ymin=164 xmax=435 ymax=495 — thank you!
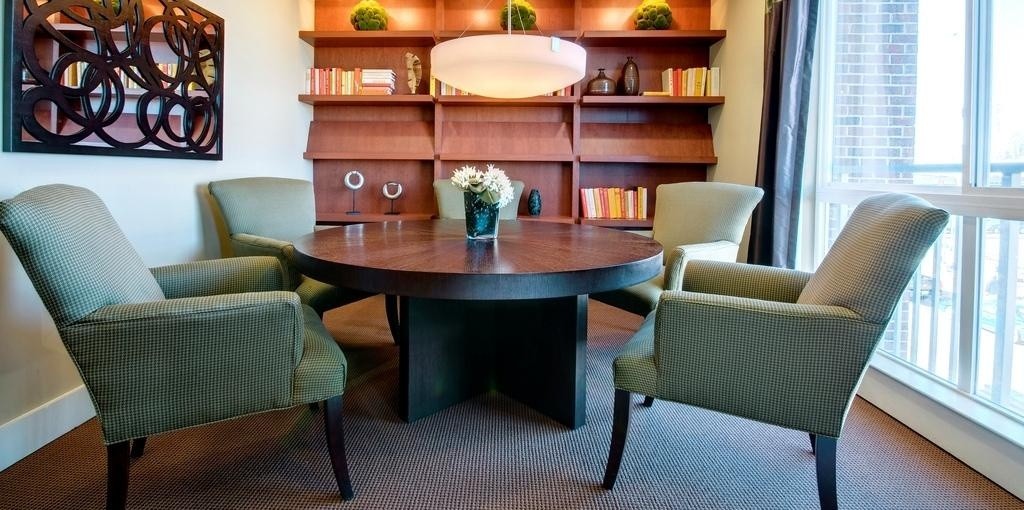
xmin=309 ymin=65 xmax=396 ymax=95
xmin=579 ymin=185 xmax=649 ymax=220
xmin=637 ymin=66 xmax=720 ymax=97
xmin=59 ymin=57 xmax=186 ymax=94
xmin=437 ymin=80 xmax=571 ymax=97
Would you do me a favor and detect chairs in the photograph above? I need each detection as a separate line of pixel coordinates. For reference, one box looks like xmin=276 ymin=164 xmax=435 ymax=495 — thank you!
xmin=431 ymin=178 xmax=527 ymax=221
xmin=206 ymin=177 xmax=400 ymax=352
xmin=597 ymin=191 xmax=948 ymax=509
xmin=0 ymin=182 xmax=361 ymax=510
xmin=587 ymin=180 xmax=766 ymax=410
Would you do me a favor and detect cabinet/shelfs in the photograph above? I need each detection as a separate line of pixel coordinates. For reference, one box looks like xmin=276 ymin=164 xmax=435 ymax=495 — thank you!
xmin=298 ymin=1 xmax=727 ymax=227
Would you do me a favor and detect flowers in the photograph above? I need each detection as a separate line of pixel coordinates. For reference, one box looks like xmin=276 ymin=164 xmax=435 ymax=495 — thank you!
xmin=452 ymin=163 xmax=515 ymax=208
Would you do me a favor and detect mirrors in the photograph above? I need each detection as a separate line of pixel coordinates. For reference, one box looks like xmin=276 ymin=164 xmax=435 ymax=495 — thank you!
xmin=3 ymin=1 xmax=225 ymax=160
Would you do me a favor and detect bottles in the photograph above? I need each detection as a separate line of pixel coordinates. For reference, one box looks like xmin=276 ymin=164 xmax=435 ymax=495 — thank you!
xmin=622 ymin=56 xmax=640 ymax=96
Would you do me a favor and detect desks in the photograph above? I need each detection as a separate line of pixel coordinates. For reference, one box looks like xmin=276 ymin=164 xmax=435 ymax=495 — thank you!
xmin=289 ymin=220 xmax=667 ymax=431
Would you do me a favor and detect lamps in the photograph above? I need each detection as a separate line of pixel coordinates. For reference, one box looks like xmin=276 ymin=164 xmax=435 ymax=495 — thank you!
xmin=432 ymin=1 xmax=586 ymax=99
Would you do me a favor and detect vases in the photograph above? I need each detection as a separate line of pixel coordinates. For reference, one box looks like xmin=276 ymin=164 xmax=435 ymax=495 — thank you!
xmin=461 ymin=192 xmax=506 ymax=242
